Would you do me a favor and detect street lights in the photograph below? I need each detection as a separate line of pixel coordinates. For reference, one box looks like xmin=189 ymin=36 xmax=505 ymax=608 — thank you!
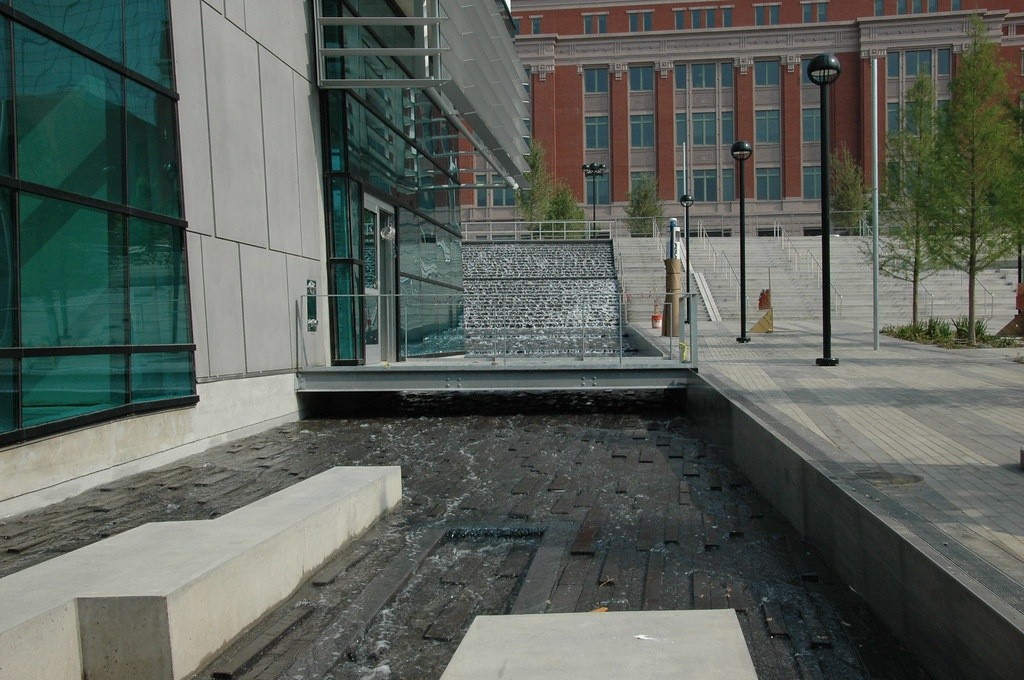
xmin=680 ymin=195 xmax=694 ymax=324
xmin=582 ymin=163 xmax=606 ymax=239
xmin=731 ymin=141 xmax=753 ymax=343
xmin=807 ymin=53 xmax=841 ymax=366
